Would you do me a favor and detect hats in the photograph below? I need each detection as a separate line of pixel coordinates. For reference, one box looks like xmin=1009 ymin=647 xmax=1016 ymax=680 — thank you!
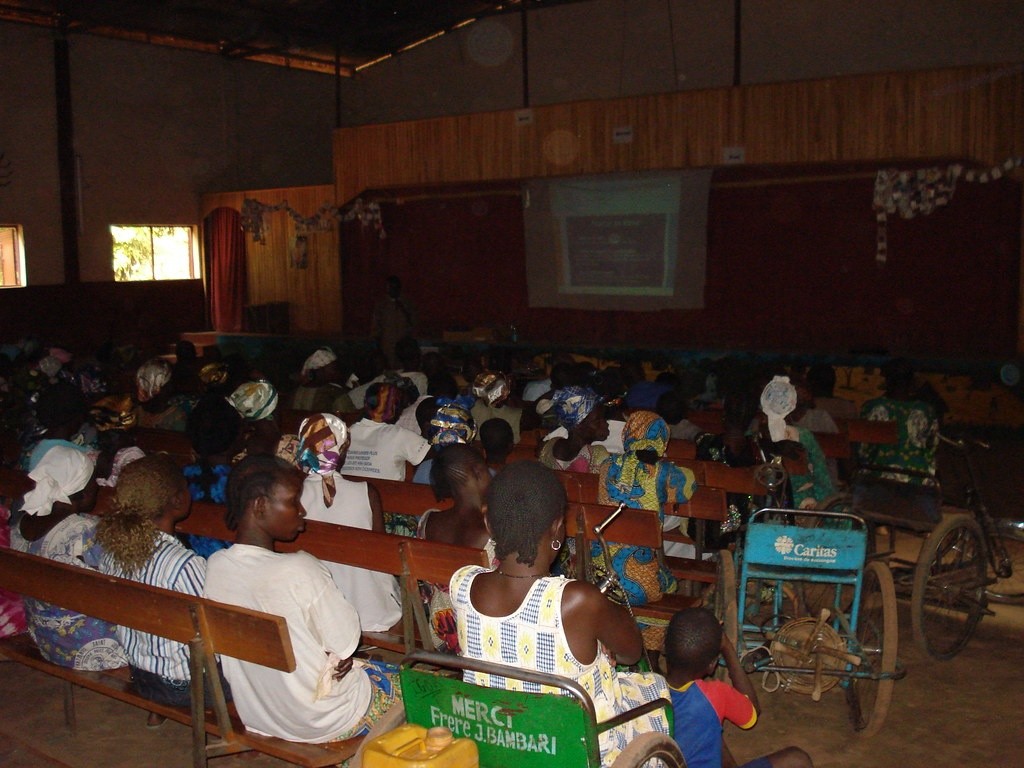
xmin=186 ymin=395 xmax=240 ymax=454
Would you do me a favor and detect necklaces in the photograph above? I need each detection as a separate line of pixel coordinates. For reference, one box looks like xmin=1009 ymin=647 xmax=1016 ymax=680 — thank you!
xmin=497 ymin=569 xmax=553 ymax=580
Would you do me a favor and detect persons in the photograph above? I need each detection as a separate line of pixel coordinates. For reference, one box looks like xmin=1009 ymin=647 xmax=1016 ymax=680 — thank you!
xmin=0 ymin=331 xmax=942 ymax=768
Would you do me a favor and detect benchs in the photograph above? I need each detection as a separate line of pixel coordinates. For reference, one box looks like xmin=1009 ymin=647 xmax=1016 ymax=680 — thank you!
xmin=0 ymin=387 xmax=900 ymax=768
